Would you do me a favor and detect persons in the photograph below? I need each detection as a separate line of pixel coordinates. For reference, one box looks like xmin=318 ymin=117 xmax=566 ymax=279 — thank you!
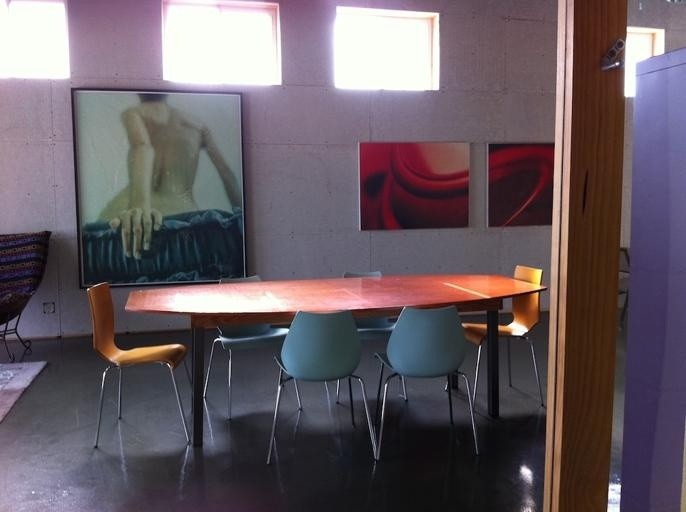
xmin=89 ymin=92 xmax=243 ymax=262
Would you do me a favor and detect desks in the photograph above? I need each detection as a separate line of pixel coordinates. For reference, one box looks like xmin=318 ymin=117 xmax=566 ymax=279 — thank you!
xmin=125 ymin=274 xmax=546 ymax=454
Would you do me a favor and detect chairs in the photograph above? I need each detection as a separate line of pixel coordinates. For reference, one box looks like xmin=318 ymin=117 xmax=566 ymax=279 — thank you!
xmin=374 ymin=305 xmax=481 ymax=461
xmin=334 ymin=271 xmax=408 ymax=405
xmin=615 ymin=246 xmax=632 ymax=330
xmin=272 ymin=310 xmax=378 ymax=465
xmin=444 ymin=267 xmax=547 ymax=411
xmin=0 ymin=231 xmax=52 ymax=361
xmin=91 ymin=281 xmax=204 ymax=450
xmin=202 ymin=276 xmax=302 ymax=420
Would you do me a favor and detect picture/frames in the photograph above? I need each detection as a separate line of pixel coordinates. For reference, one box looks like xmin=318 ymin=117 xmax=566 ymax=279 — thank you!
xmin=485 ymin=141 xmax=554 ymax=229
xmin=358 ymin=140 xmax=472 ymax=232
xmin=69 ymin=85 xmax=249 ymax=290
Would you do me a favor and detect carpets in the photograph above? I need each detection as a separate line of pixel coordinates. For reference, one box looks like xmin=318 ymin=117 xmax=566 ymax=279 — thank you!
xmin=0 ymin=360 xmax=48 ymax=425
